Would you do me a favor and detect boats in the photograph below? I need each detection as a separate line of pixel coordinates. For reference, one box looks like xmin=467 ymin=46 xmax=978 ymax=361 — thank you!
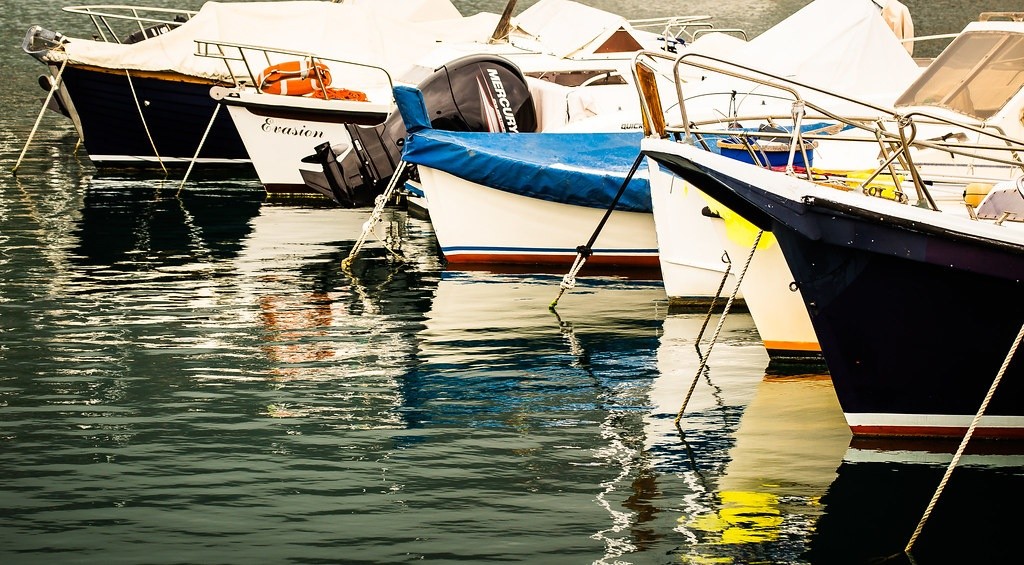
xmin=0 ymin=0 xmax=1023 ymax=473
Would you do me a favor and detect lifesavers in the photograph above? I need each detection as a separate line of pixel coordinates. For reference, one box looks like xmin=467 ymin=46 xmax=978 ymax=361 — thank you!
xmin=256 ymin=60 xmax=334 ymax=96
